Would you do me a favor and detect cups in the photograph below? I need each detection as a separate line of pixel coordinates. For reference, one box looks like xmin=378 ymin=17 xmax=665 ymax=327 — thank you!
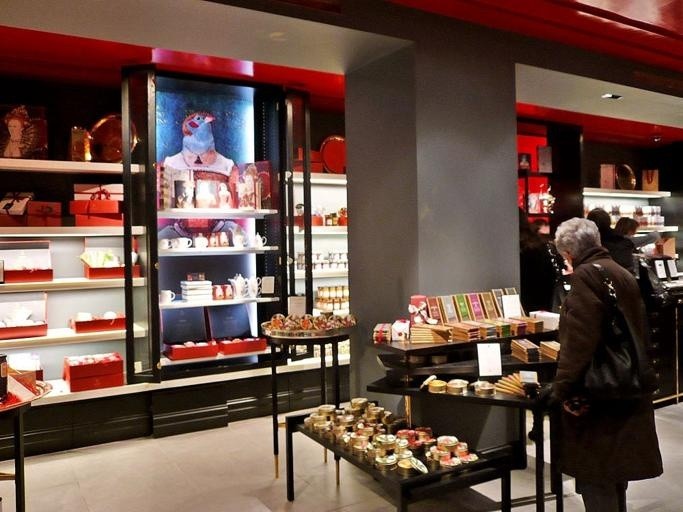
xmin=157 ymin=236 xmax=192 ymax=249
xmin=160 ymin=289 xmax=176 ymax=304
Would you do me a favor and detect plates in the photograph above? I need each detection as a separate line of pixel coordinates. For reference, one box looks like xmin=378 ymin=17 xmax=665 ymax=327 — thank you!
xmin=616 ymin=163 xmax=637 ymax=190
xmin=89 ymin=113 xmax=136 ymax=162
xmin=320 ymin=134 xmax=345 ymax=174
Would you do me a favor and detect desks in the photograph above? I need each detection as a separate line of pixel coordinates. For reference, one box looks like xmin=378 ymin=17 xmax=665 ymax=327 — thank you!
xmin=1 ymin=374 xmax=52 ymax=510
xmin=284 ymin=407 xmax=512 ymax=511
xmin=264 ymin=312 xmax=352 ymax=484
xmin=367 ymin=316 xmax=565 ymax=511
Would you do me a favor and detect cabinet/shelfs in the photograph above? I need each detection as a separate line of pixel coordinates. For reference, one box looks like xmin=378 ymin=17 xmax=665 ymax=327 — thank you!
xmin=516 ymin=113 xmax=683 ymax=421
xmin=0 ymin=61 xmax=347 ymax=459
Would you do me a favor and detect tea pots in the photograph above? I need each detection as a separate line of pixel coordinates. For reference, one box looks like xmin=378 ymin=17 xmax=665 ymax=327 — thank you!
xmin=224 ymin=226 xmax=267 ymax=249
xmin=227 ymin=273 xmax=262 ymax=299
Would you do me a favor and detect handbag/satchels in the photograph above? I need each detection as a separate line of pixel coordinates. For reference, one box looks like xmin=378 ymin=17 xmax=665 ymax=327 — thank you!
xmin=577 ymin=262 xmax=660 ymax=402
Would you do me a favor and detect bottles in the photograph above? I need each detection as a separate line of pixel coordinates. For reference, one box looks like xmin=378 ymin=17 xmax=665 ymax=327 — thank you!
xmin=330 ymin=213 xmax=338 ymax=225
xmin=295 ymin=251 xmax=348 ymax=317
xmin=0 ymin=354 xmax=8 ymax=402
xmin=295 ymin=203 xmax=305 ymax=226
xmin=324 ymin=214 xmax=331 ymax=226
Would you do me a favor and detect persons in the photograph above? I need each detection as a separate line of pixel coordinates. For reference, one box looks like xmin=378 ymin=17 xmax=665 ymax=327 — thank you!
xmin=528 ymin=205 xmax=648 ymax=276
xmin=549 ymin=217 xmax=663 ymax=511
xmin=0 ymin=106 xmax=39 ymax=159
xmin=518 ymin=206 xmax=558 ymax=444
xmin=613 ymin=217 xmax=664 ymax=299
